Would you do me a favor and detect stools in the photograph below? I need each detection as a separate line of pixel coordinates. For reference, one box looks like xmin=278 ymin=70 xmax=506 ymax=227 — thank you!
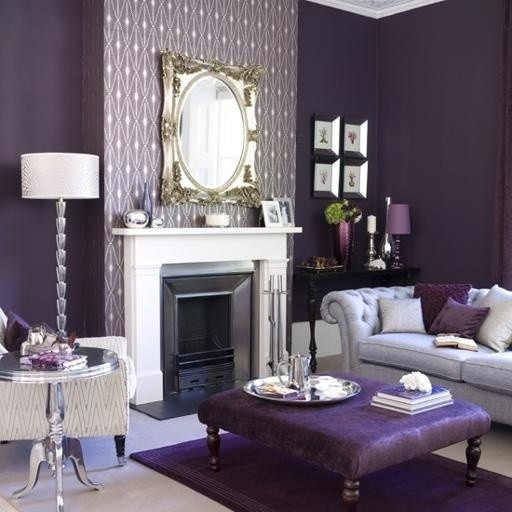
xmin=197 ymin=370 xmax=490 ymax=511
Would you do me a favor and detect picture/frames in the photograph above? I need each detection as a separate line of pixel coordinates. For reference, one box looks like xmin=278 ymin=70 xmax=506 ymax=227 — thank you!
xmin=344 ymin=119 xmax=368 ymax=160
xmin=259 ymin=201 xmax=283 ymax=227
xmin=344 ymin=161 xmax=370 ymax=199
xmin=310 ymin=159 xmax=340 ymax=199
xmin=274 ymin=197 xmax=295 ymax=226
xmin=311 ymin=112 xmax=340 ymax=157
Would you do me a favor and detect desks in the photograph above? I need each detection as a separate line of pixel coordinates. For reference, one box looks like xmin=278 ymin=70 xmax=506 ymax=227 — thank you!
xmin=296 ymin=262 xmax=421 ymax=373
xmin=1 ymin=348 xmax=119 ymax=511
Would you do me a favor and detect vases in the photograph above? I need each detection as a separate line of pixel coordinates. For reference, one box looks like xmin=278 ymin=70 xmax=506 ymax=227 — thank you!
xmin=378 ymin=196 xmax=396 ymax=267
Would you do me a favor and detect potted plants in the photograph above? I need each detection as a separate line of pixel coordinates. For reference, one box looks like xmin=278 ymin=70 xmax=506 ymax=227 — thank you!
xmin=324 ymin=199 xmax=362 ymax=263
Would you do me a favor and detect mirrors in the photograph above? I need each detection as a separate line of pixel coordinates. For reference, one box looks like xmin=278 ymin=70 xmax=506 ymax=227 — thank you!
xmin=158 ymin=49 xmax=263 ymax=206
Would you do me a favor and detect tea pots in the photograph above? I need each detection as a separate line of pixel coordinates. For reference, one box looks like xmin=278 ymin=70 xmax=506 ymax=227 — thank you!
xmin=277 ymin=351 xmax=312 ymax=390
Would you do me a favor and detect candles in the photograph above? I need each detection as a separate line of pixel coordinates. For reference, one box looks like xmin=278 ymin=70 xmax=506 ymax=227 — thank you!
xmin=368 ymin=215 xmax=376 ymax=232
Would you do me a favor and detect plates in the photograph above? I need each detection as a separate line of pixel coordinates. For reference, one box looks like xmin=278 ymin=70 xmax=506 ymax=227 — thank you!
xmin=242 ymin=375 xmax=362 ymax=405
xmin=296 ymin=265 xmax=343 ymax=272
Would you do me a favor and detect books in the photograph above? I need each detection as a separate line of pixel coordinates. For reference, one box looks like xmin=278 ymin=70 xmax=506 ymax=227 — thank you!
xmin=435 ymin=334 xmax=478 ymax=352
xmin=371 ymin=382 xmax=455 ymax=416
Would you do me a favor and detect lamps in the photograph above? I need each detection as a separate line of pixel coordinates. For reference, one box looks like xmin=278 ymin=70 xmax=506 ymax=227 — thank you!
xmin=18 ymin=152 xmax=100 ymax=344
xmin=386 ymin=204 xmax=411 ymax=267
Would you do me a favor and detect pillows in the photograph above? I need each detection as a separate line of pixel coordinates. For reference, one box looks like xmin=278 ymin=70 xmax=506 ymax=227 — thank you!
xmin=413 ymin=282 xmax=472 ymax=333
xmin=427 ymin=295 xmax=490 ymax=345
xmin=378 ymin=297 xmax=428 ymax=334
xmin=476 ymin=284 xmax=512 ymax=352
xmin=6 ymin=310 xmax=33 ymax=341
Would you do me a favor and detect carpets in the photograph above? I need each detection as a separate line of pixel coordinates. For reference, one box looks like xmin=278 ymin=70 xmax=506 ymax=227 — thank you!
xmin=130 ymin=433 xmax=512 ymax=511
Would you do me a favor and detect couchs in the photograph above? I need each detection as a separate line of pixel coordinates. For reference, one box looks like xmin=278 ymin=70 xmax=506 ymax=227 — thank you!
xmin=319 ymin=284 xmax=512 ymax=427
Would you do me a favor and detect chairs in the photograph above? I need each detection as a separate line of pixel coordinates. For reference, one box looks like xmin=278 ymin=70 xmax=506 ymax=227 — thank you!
xmin=0 ymin=304 xmax=137 ymax=467
